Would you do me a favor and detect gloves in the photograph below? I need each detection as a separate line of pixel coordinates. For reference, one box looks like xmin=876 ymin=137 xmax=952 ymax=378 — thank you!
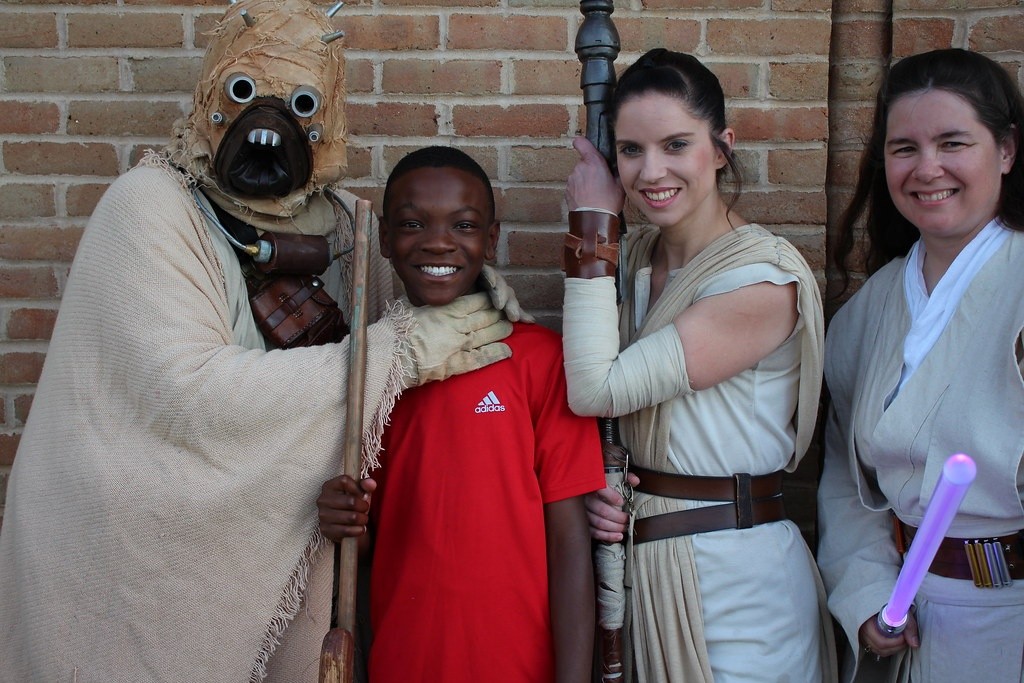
xmin=480 ymin=264 xmax=536 ymax=324
xmin=398 ymin=291 xmax=513 ymax=391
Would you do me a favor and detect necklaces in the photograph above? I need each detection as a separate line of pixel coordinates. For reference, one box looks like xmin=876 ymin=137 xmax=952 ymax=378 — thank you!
xmin=190 ymin=185 xmax=356 ymax=274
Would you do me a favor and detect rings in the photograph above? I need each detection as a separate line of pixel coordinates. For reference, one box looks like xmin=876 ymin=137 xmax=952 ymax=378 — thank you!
xmin=864 ymin=645 xmax=872 ymax=655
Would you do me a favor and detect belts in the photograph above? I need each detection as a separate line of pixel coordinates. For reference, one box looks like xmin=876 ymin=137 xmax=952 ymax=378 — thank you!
xmin=890 ymin=509 xmax=1024 ymax=580
xmin=620 ymin=464 xmax=786 ymax=546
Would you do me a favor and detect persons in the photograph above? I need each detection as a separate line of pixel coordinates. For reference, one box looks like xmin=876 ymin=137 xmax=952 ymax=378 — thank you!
xmin=816 ymin=48 xmax=1024 ymax=683
xmin=0 ymin=0 xmax=535 ymax=683
xmin=563 ymin=48 xmax=839 ymax=683
xmin=318 ymin=145 xmax=608 ymax=683
